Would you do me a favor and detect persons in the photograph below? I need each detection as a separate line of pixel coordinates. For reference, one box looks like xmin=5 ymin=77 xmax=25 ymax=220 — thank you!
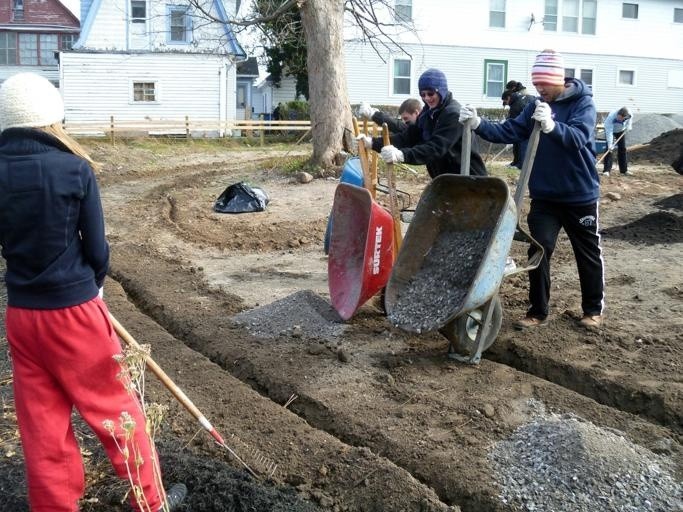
xmin=501 ymin=80 xmax=531 ymax=166
xmin=0 ymin=73 xmax=188 ymax=511
xmin=355 ymin=98 xmax=422 ymax=133
xmin=458 ymin=47 xmax=606 ymax=327
xmin=604 ymin=107 xmax=633 ymax=177
xmin=356 ymin=68 xmax=518 ymax=275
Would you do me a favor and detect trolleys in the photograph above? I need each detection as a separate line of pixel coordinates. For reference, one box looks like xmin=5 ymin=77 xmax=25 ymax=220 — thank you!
xmin=381 ymin=106 xmax=542 ymax=368
xmin=326 ymin=115 xmax=401 ymax=322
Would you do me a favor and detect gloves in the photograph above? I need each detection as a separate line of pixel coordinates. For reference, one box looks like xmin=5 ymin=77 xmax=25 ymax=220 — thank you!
xmin=458 ymin=105 xmax=481 ymax=131
xmin=352 ymin=134 xmax=374 ymax=153
xmin=531 ymin=100 xmax=556 ymax=133
xmin=358 ymin=102 xmax=379 ymax=122
xmin=624 ymin=128 xmax=632 ymax=134
xmin=608 ymin=146 xmax=615 ymax=154
xmin=381 ymin=145 xmax=406 ymax=165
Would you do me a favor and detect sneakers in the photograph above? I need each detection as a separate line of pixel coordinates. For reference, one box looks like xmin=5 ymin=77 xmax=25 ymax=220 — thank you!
xmin=159 ymin=484 xmax=187 ymax=512
xmin=512 ymin=318 xmax=548 ymax=331
xmin=579 ymin=316 xmax=604 ymax=329
xmin=603 ymin=172 xmax=610 ymax=178
xmin=621 ymin=171 xmax=634 ymax=177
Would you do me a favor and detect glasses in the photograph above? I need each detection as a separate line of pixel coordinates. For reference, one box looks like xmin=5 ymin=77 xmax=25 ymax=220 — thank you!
xmin=421 ymin=93 xmax=435 ymax=97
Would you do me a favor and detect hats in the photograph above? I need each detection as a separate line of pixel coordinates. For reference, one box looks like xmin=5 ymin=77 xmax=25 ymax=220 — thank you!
xmin=531 ymin=50 xmax=565 ymax=86
xmin=1 ymin=73 xmax=65 ymax=135
xmin=419 ymin=69 xmax=448 ymax=105
